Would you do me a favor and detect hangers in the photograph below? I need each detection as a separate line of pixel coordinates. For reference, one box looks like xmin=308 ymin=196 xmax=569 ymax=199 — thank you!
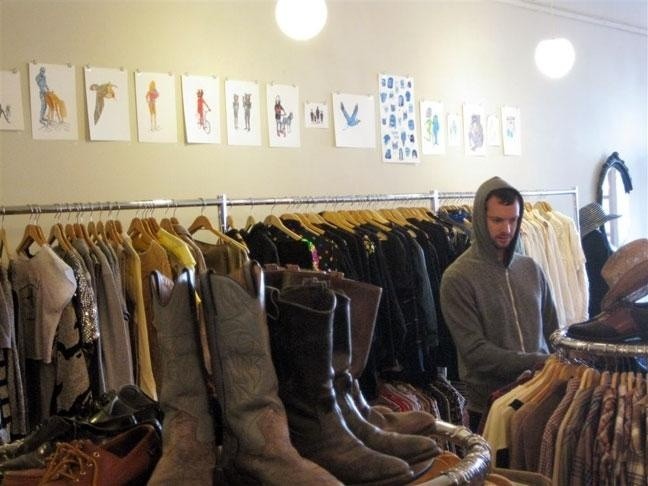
xmin=504 ymin=327 xmax=648 ymax=431
xmin=1 ymin=190 xmax=554 ymax=264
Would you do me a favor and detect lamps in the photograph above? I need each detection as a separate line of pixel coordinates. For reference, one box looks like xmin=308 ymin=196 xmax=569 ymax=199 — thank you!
xmin=531 ymin=0 xmax=576 ymax=82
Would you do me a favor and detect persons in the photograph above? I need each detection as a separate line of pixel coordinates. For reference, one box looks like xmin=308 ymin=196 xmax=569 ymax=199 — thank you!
xmin=232 ymin=94 xmax=240 ymax=129
xmin=197 ymin=88 xmax=212 ymax=129
xmin=35 ymin=65 xmax=54 ymax=128
xmin=146 ymin=80 xmax=161 ymax=130
xmin=381 ymin=75 xmax=418 ymax=159
xmin=309 ymin=104 xmax=324 ymax=125
xmin=431 ymin=115 xmax=439 ymax=146
xmin=241 ymin=93 xmax=252 ymax=131
xmin=274 ymin=93 xmax=287 ymax=135
xmin=440 ymin=175 xmax=559 ymax=434
xmin=425 ymin=107 xmax=433 ymax=140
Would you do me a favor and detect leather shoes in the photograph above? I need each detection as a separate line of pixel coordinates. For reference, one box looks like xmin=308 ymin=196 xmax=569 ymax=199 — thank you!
xmin=566 ymin=301 xmax=648 ymax=342
xmin=0 ymin=384 xmax=163 ymax=486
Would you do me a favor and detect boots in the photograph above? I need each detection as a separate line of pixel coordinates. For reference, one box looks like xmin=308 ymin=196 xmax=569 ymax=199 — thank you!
xmin=148 ymin=268 xmax=217 ymax=486
xmin=200 ymin=260 xmax=344 ymax=486
xmin=265 ymin=263 xmax=442 ymax=486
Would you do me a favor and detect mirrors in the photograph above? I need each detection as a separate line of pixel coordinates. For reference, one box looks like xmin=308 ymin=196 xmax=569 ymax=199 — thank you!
xmin=597 ymin=151 xmax=637 ymax=253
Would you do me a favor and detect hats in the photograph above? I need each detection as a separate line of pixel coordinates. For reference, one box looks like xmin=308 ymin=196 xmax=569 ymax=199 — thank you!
xmin=600 ymin=239 xmax=648 ymax=311
xmin=580 ymin=202 xmax=622 ymax=238
